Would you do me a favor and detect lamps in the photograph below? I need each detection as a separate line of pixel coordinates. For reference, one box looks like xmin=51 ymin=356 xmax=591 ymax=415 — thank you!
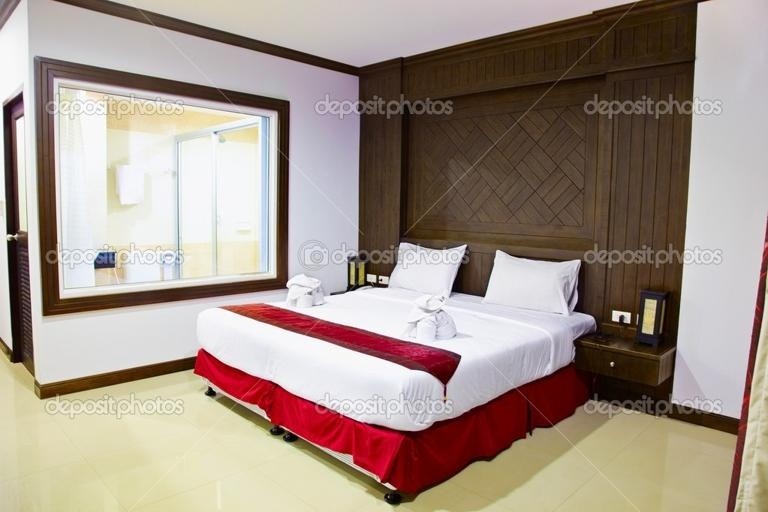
xmin=632 ymin=288 xmax=671 ymax=351
xmin=346 ymin=255 xmax=369 ymax=292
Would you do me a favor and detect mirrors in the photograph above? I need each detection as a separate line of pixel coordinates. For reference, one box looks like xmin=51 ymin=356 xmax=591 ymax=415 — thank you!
xmin=34 ymin=55 xmax=290 ymax=317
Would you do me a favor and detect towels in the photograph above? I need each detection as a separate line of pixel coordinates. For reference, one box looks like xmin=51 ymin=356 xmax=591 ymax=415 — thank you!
xmin=117 ymin=164 xmax=147 ymax=207
xmin=408 ymin=293 xmax=456 ymax=343
xmin=285 ymin=273 xmax=327 ymax=312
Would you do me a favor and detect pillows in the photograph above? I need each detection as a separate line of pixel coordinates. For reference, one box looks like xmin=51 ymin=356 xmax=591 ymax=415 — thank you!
xmin=388 ymin=241 xmax=468 ymax=299
xmin=482 ymin=249 xmax=581 ymax=316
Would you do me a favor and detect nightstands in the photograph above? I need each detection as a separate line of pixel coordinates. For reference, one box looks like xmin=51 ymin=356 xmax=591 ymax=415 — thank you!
xmin=574 ymin=335 xmax=676 ymax=394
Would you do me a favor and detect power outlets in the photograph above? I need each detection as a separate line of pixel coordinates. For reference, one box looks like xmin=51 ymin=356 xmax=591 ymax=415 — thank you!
xmin=612 ymin=310 xmax=632 ymax=325
xmin=366 ymin=273 xmax=390 ymax=285
